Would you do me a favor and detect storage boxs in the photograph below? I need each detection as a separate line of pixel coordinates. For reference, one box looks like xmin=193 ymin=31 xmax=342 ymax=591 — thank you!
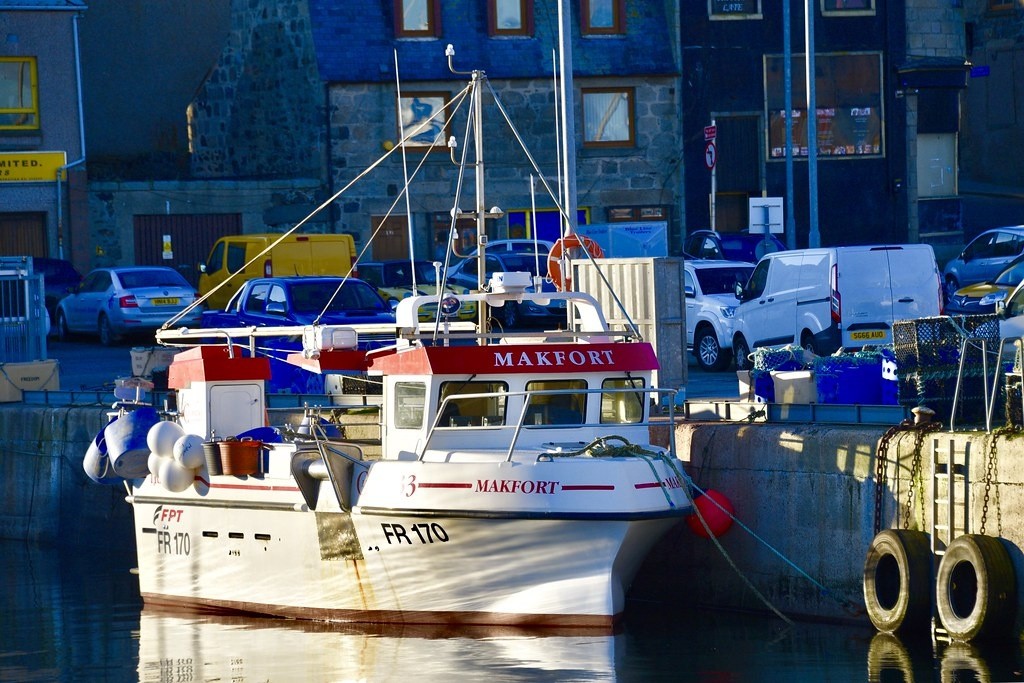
xmin=737 ymin=370 xmax=755 ymax=402
xmin=769 ymin=371 xmax=818 ymax=404
xmin=131 ymin=351 xmax=180 ymax=377
xmin=0 ymin=358 xmax=60 ymax=403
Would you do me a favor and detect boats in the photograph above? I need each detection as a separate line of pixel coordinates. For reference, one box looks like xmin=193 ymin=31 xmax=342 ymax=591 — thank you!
xmin=117 ymin=41 xmax=692 ymax=627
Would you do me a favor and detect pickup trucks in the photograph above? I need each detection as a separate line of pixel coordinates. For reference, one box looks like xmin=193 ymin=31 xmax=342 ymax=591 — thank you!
xmin=202 ymin=275 xmax=400 ymax=346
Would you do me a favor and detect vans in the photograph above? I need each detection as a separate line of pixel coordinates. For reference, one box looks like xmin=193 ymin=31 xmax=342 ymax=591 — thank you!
xmin=197 ymin=233 xmax=359 ymax=310
xmin=734 ymin=243 xmax=944 ymax=371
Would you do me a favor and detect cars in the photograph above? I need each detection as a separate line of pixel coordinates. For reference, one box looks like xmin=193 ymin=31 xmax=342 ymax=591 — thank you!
xmin=359 ymin=258 xmax=478 ymax=321
xmin=55 ymin=265 xmax=206 ymax=343
xmin=683 ymin=259 xmax=758 ymax=371
xmin=969 ymin=278 xmax=1024 ymax=359
xmin=442 ymin=250 xmax=569 ymax=328
xmin=945 ymin=225 xmax=1024 ymax=296
xmin=34 ymin=256 xmax=84 ymax=301
xmin=468 ymin=240 xmax=557 ymax=256
xmin=700 ymin=229 xmax=789 ymax=263
xmin=953 ymin=263 xmax=1023 ymax=311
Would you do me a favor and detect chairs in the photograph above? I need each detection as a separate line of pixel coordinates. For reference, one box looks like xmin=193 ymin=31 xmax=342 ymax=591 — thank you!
xmin=438 ymin=402 xmax=460 ymax=427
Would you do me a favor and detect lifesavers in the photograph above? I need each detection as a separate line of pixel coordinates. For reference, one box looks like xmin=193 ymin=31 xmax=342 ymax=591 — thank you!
xmin=547 ymin=235 xmax=605 ymax=290
xmin=936 ymin=533 xmax=1015 ymax=643
xmin=862 ymin=529 xmax=931 ymax=634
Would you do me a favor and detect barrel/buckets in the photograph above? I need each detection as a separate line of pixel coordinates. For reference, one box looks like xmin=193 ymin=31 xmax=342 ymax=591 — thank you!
xmin=294 ymin=415 xmax=342 ymax=449
xmin=753 ymin=343 xmax=1023 ymax=423
xmin=102 ymin=406 xmax=160 ymax=479
xmin=217 ymin=437 xmax=259 ymax=475
xmin=83 ymin=415 xmax=126 ymax=486
xmin=237 ymin=426 xmax=283 ymax=471
xmin=201 ymin=436 xmax=237 ymax=476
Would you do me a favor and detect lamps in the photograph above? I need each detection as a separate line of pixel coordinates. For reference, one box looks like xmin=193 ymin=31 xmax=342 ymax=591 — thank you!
xmin=441 ymin=293 xmax=461 ymax=334
